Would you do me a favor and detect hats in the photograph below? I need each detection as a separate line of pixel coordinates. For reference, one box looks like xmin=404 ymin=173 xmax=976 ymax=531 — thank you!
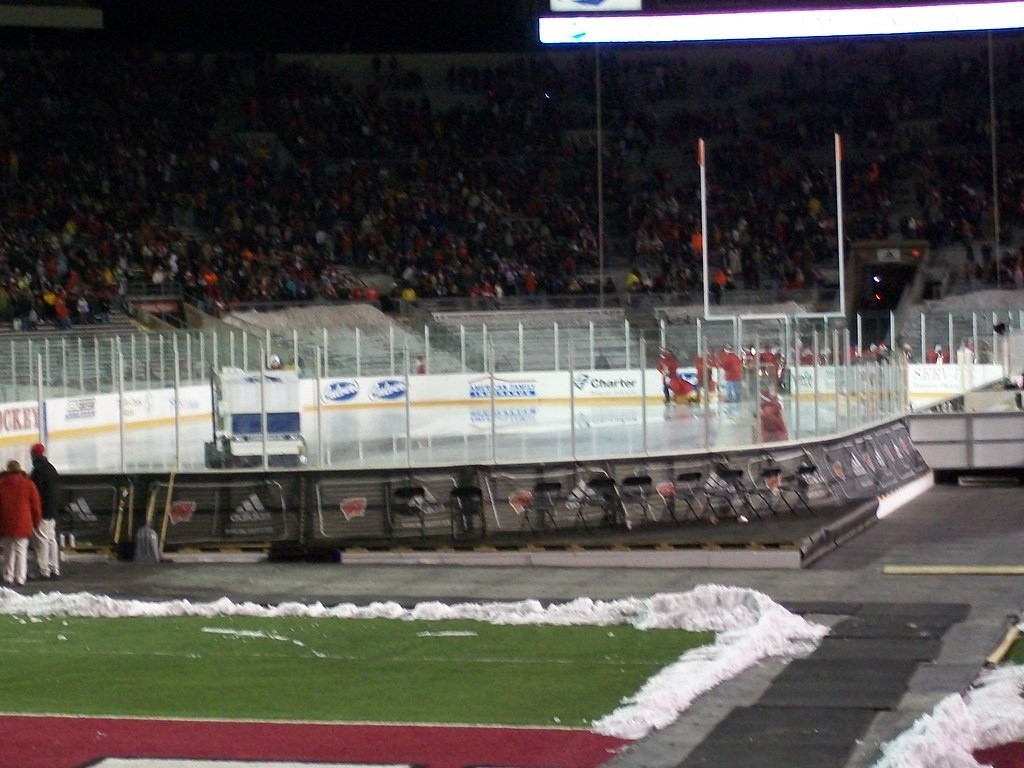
xmin=32 ymin=444 xmax=45 ymax=453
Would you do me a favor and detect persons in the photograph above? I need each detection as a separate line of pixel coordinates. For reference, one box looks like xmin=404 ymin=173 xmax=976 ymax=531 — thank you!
xmin=414 ymin=354 xmax=425 ymax=373
xmin=759 ymin=387 xmax=788 ymax=443
xmin=978 ymin=339 xmax=993 ymax=364
xmin=264 ymin=349 xmax=304 ymax=381
xmin=0 ymin=40 xmax=1024 ymax=326
xmin=957 ymin=337 xmax=973 ymax=365
xmin=27 ymin=443 xmax=60 ymax=580
xmin=657 ymin=343 xmax=948 ymax=402
xmin=0 ymin=460 xmax=42 ymax=587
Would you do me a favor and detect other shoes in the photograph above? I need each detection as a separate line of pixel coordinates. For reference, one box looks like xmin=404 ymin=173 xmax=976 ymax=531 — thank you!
xmin=0 ymin=573 xmax=12 ymax=586
xmin=27 ymin=571 xmax=50 ymax=580
xmin=13 ymin=578 xmax=24 ymax=588
xmin=50 ymin=571 xmax=59 ymax=577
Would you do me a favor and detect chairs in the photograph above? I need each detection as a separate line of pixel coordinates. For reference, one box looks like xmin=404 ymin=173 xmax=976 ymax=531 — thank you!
xmin=385 ymin=487 xmax=426 ymax=540
xmin=449 ymin=487 xmax=488 ymax=540
xmin=664 ymin=471 xmax=720 ymax=525
xmin=571 ymin=477 xmax=623 ymax=537
xmin=773 ymin=464 xmax=819 ymax=517
xmin=744 ymin=469 xmax=794 ymax=517
xmin=519 ymin=482 xmax=563 ymax=537
xmin=711 ymin=470 xmax=762 ymax=517
xmin=610 ymin=475 xmax=659 ymax=535
